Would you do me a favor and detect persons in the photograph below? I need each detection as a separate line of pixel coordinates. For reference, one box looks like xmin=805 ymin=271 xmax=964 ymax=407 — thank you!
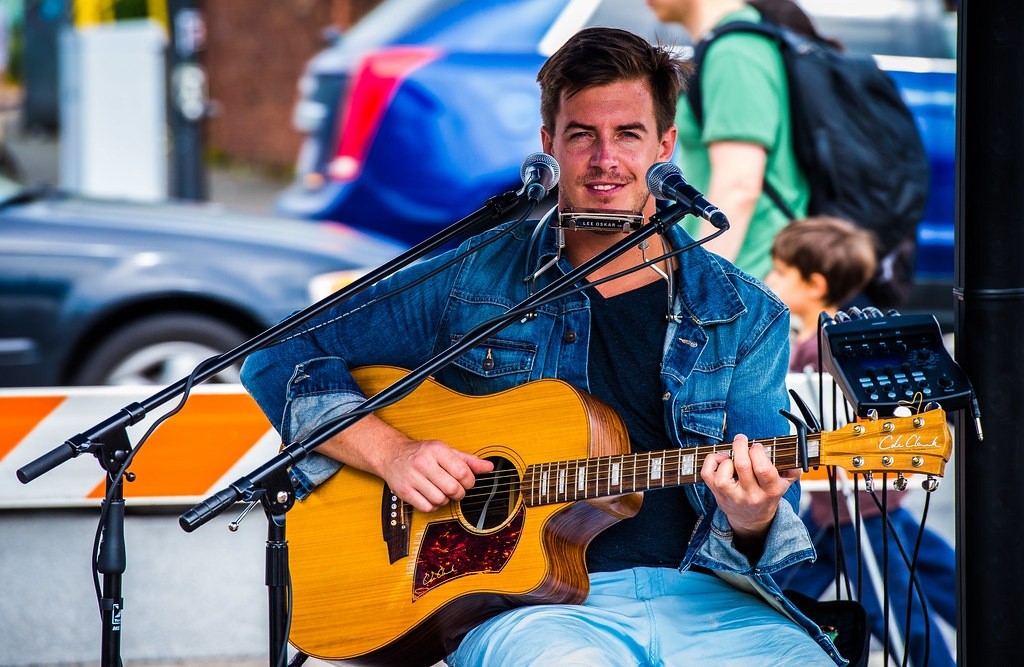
xmin=763 ymin=217 xmax=956 ymax=667
xmin=647 ymin=0 xmax=956 ymax=631
xmin=237 ymin=27 xmax=853 ymax=667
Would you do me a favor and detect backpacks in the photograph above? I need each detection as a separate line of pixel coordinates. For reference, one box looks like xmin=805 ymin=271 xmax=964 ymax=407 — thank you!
xmin=686 ymin=21 xmax=931 ymax=268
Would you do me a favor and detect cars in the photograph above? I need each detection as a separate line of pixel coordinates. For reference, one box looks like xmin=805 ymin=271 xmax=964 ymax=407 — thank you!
xmin=271 ymin=0 xmax=959 ymax=299
xmin=0 ymin=139 xmax=419 ymax=386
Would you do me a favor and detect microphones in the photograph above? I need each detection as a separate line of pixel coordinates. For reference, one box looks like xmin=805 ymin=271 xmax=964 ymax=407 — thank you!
xmin=519 ymin=152 xmax=561 ymax=208
xmin=646 ymin=161 xmax=731 ymax=230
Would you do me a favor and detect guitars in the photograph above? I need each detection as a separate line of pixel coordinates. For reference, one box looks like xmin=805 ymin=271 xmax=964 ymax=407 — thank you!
xmin=278 ymin=363 xmax=955 ymax=666
